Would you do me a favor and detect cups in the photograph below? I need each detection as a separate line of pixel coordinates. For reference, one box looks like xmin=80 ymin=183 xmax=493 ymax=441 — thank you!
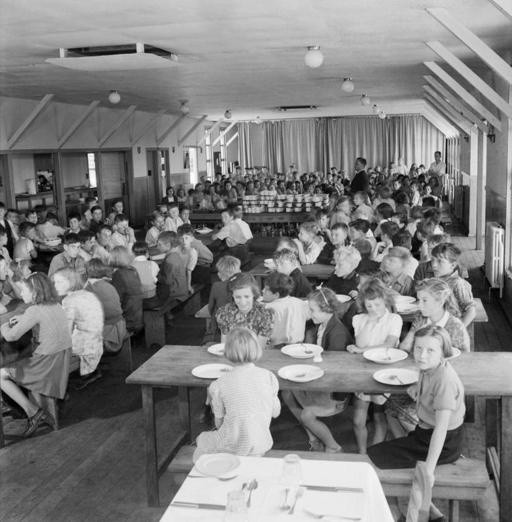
xmin=279 ymin=452 xmax=306 ymax=487
xmin=223 ymin=491 xmax=249 ymax=522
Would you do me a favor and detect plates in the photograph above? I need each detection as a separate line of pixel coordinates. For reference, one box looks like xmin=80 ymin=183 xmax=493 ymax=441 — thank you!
xmin=334 ymin=293 xmax=352 ymax=303
xmin=447 ymin=346 xmax=461 ymax=360
xmin=196 ymin=225 xmax=212 ymax=235
xmin=276 ymin=362 xmax=328 ymax=384
xmin=208 ymin=340 xmax=227 ymax=357
xmin=363 ymin=347 xmax=408 ymax=364
xmin=373 ymin=366 xmax=422 ymax=388
xmin=191 ymin=362 xmax=237 ymax=381
xmin=394 ymin=292 xmax=419 ymax=316
xmin=262 ymin=256 xmax=274 ymax=268
xmin=193 ymin=453 xmax=242 ymax=478
xmin=278 ymin=341 xmax=325 ymax=359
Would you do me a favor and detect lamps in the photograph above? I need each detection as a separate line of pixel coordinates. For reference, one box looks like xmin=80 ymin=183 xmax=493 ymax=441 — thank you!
xmin=179 ymin=101 xmax=261 ymax=125
xmin=342 ymin=77 xmax=354 ymax=91
xmin=360 ymin=92 xmax=387 ymax=119
xmin=108 ymin=90 xmax=120 ymax=103
xmin=304 ymin=46 xmax=323 ymax=67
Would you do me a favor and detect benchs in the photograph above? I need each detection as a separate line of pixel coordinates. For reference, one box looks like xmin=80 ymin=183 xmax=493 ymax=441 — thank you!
xmin=165 ymin=443 xmax=489 ymax=521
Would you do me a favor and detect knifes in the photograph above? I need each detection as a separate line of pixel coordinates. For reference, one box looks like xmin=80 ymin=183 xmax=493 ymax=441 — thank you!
xmin=168 ymin=500 xmax=226 ymax=514
xmin=300 ymin=484 xmax=365 ymax=495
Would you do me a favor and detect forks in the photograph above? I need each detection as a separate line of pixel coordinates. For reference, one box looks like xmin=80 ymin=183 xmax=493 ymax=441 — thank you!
xmin=183 ymin=473 xmax=240 ymax=485
xmin=288 ymin=488 xmax=304 ymax=515
xmin=304 ymin=509 xmax=362 ymax=522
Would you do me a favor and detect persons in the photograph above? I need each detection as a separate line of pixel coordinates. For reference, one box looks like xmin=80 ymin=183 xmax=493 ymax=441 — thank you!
xmin=149 ymin=146 xmax=449 ymax=213
xmin=366 ymin=325 xmax=467 ymax=522
xmin=0 ymin=194 xmax=254 ymax=435
xmin=191 ymin=185 xmax=471 ymax=464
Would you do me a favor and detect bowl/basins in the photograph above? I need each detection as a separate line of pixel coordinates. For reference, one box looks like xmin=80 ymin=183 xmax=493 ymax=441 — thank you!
xmin=43 ymin=237 xmax=63 ymax=247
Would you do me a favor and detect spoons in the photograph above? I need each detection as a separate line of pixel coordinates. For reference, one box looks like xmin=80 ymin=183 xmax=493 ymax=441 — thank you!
xmin=279 ymin=486 xmax=289 ymax=514
xmin=242 ymin=476 xmax=261 ymax=509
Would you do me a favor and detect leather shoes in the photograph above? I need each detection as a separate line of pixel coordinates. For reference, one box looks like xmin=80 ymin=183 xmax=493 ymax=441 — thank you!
xmin=23 ymin=408 xmax=47 ymax=437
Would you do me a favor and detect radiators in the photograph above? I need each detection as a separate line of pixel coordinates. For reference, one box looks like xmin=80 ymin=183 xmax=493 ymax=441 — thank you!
xmin=484 ymin=219 xmax=505 ymax=290
